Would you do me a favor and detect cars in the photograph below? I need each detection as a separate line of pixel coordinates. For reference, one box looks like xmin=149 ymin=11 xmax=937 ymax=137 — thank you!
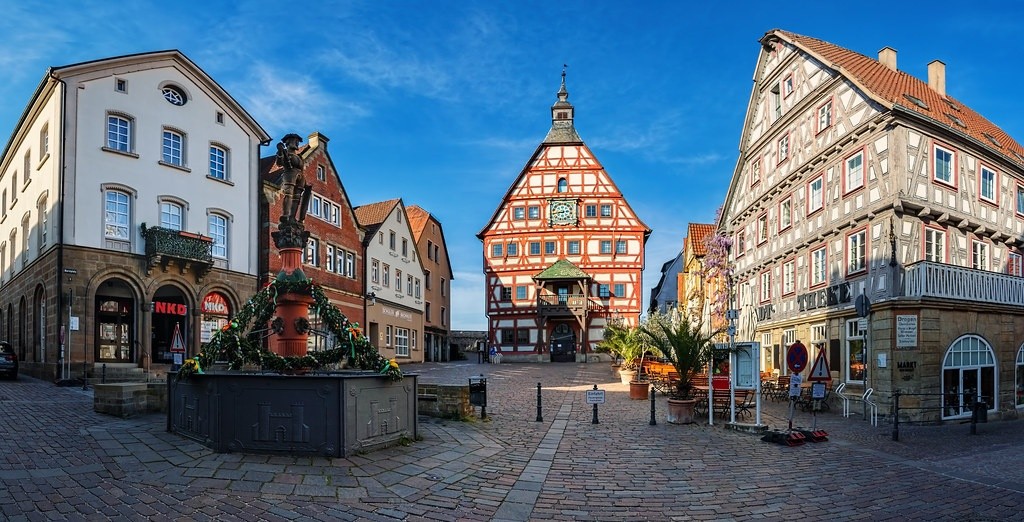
xmin=0 ymin=341 xmax=18 ymax=379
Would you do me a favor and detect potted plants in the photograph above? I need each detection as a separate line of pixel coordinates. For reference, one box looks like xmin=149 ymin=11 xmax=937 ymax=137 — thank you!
xmin=638 ymin=318 xmax=752 ymax=425
xmin=629 ymin=330 xmax=658 ymax=400
xmin=596 ymin=324 xmax=631 ymax=379
xmin=600 ymin=324 xmax=663 ymax=385
xmin=594 ymin=322 xmax=627 ymax=371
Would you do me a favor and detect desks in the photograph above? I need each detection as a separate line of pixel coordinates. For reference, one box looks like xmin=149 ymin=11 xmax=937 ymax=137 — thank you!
xmin=797 ymin=382 xmax=830 ymax=408
xmin=763 ymin=377 xmax=780 ymax=386
xmin=694 ymin=385 xmax=748 ymax=418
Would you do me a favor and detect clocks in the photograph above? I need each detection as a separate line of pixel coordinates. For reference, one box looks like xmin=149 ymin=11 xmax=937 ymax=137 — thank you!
xmin=547 ymin=197 xmax=584 ymax=228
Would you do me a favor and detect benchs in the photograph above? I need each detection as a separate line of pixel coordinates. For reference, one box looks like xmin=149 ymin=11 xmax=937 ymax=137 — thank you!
xmin=761 ymin=372 xmax=834 ymax=416
xmin=634 ymin=360 xmax=756 ymax=421
